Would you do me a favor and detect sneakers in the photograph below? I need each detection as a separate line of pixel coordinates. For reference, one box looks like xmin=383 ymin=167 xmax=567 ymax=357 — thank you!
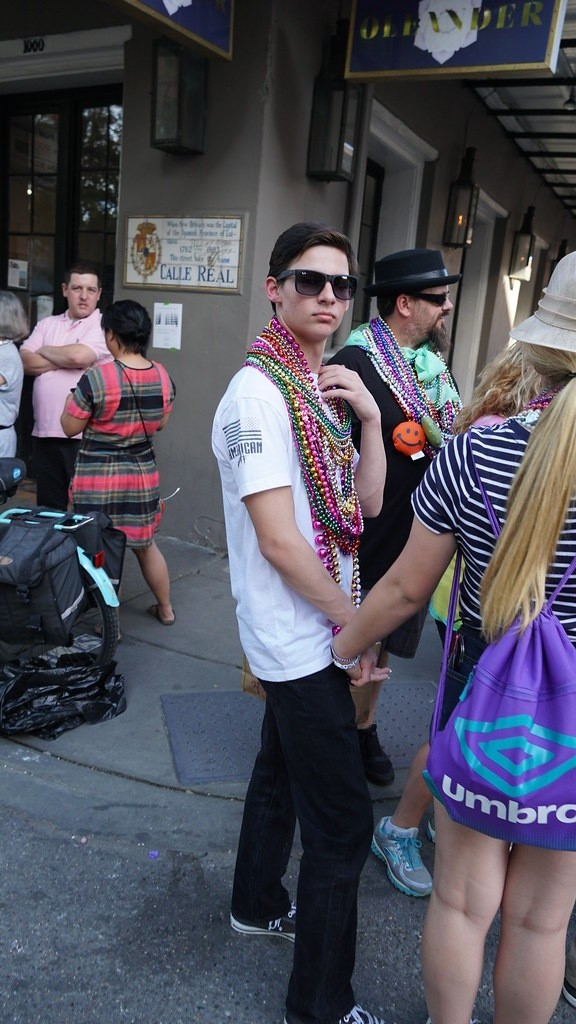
xmin=425 ymin=818 xmax=436 ymax=843
xmin=357 ymin=724 xmax=395 ymax=785
xmin=370 ymin=816 xmax=432 ymax=897
xmin=283 ymin=1004 xmax=395 ymax=1024
xmin=230 ymin=900 xmax=296 ymax=942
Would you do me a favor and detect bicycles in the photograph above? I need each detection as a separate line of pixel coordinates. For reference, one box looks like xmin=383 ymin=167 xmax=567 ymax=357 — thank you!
xmin=0 ymin=457 xmax=119 ymax=675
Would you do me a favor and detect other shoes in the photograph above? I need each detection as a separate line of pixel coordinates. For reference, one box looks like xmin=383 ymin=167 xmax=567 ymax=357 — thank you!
xmin=562 ymin=977 xmax=576 ymax=1008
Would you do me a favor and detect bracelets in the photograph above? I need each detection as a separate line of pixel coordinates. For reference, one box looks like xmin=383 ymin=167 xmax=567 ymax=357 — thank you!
xmin=330 ymin=641 xmax=355 ymax=662
xmin=332 ymin=653 xmax=361 ymax=670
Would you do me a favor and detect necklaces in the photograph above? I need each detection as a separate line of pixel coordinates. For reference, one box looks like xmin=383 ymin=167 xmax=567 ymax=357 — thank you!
xmin=242 ymin=317 xmax=364 ymax=639
xmin=364 ymin=312 xmax=464 ymax=457
xmin=522 ymin=379 xmax=569 ymax=412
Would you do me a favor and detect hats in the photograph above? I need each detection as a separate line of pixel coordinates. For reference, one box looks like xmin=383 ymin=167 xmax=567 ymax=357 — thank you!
xmin=509 ymin=251 xmax=576 ymax=352
xmin=363 ymin=249 xmax=463 ymax=297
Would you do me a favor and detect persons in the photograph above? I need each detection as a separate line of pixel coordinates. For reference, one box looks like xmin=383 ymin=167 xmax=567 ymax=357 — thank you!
xmin=19 ymin=262 xmax=115 ymax=513
xmin=0 ymin=291 xmax=30 ymax=461
xmin=370 ymin=329 xmax=548 ymax=898
xmin=61 ymin=299 xmax=175 ymax=641
xmin=329 ymin=245 xmax=576 ymax=1024
xmin=325 ymin=248 xmax=465 ymax=788
xmin=210 ymin=222 xmax=387 ymax=1024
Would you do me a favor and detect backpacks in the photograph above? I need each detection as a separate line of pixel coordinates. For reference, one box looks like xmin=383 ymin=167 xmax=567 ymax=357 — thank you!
xmin=422 ymin=429 xmax=576 ymax=851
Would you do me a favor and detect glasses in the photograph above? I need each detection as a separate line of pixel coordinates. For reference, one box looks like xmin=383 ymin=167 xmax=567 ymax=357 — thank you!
xmin=276 ymin=269 xmax=358 ymax=300
xmin=397 ymin=290 xmax=450 ymax=307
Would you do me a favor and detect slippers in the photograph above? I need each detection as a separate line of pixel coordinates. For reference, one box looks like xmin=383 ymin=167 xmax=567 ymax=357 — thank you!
xmin=148 ymin=604 xmax=175 ymax=625
xmin=93 ymin=622 xmax=124 ymax=643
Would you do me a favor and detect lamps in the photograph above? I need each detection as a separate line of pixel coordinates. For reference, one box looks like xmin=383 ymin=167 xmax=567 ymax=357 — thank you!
xmin=506 ymin=204 xmax=537 ymax=281
xmin=444 ymin=146 xmax=481 ymax=248
xmin=151 ymin=34 xmax=206 ymax=158
xmin=305 ymin=31 xmax=365 ymax=184
xmin=563 ymin=87 xmax=576 ymax=111
xmin=549 ymin=239 xmax=568 ymax=281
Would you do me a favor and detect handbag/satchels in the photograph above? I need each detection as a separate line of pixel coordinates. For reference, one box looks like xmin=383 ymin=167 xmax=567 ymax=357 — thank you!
xmin=0 ymin=506 xmax=127 ymax=646
